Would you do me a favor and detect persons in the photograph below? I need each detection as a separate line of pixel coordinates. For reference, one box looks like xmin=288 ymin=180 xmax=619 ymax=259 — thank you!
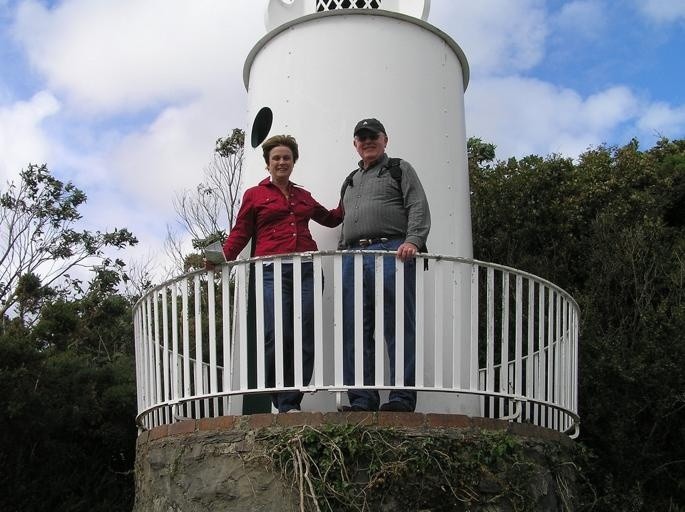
xmin=201 ymin=134 xmax=345 ymax=414
xmin=335 ymin=116 xmax=434 ymax=413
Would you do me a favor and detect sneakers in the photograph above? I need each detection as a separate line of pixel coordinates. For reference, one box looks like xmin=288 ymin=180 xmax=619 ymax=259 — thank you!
xmin=379 ymin=401 xmax=412 ymax=412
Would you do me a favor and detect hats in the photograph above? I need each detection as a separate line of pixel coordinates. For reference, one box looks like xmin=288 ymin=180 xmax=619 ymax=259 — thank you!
xmin=354 ymin=118 xmax=387 ymax=136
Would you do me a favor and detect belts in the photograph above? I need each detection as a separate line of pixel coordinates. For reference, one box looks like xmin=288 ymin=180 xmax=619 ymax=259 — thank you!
xmin=349 ymin=234 xmax=402 ymax=248
